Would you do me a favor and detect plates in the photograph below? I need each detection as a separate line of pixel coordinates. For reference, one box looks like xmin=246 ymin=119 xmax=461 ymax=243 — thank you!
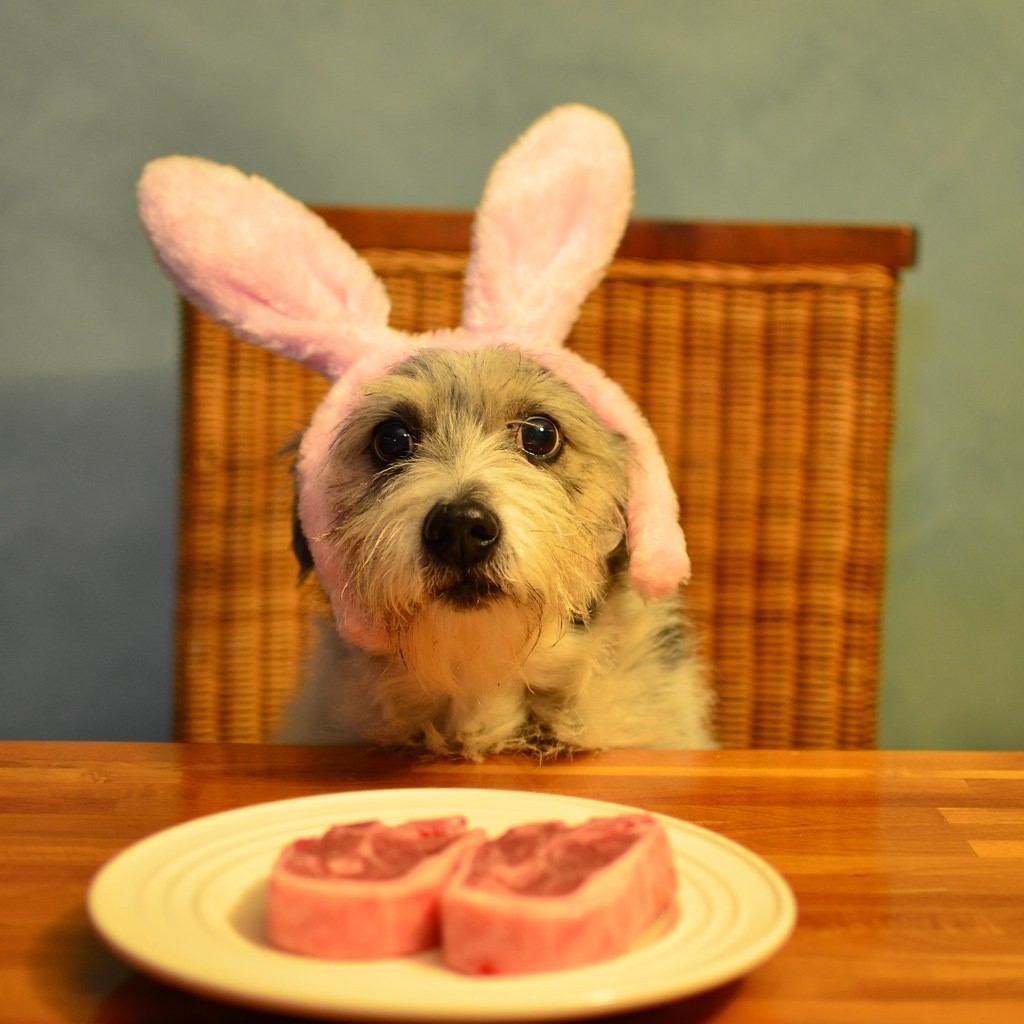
xmin=86 ymin=787 xmax=799 ymax=1021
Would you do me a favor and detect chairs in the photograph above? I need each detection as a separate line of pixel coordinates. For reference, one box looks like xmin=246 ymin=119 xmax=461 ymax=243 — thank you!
xmin=172 ymin=210 xmax=919 ymax=751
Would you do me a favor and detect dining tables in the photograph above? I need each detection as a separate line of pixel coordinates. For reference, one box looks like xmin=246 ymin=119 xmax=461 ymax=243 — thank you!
xmin=0 ymin=738 xmax=1024 ymax=1024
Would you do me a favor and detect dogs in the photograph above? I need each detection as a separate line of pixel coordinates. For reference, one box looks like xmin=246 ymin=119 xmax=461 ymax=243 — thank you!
xmin=268 ymin=344 xmax=723 ymax=764
